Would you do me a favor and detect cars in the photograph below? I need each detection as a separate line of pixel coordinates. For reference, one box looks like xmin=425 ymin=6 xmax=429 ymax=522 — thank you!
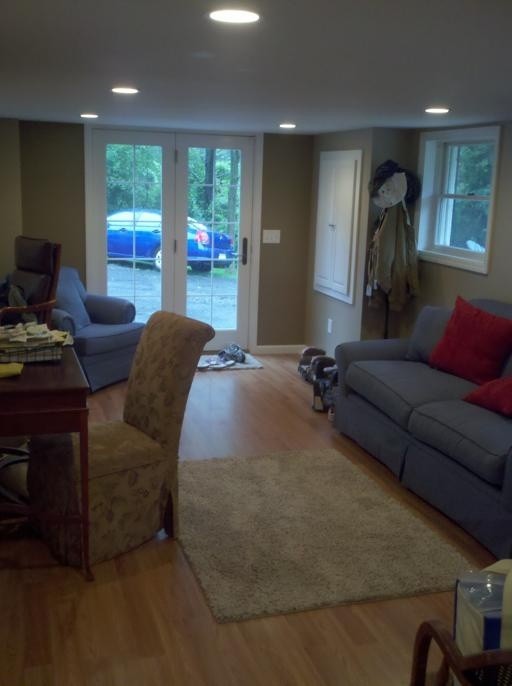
xmin=106 ymin=207 xmax=235 ymax=276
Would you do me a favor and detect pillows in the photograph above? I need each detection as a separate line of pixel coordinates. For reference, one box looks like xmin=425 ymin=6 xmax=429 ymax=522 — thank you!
xmin=428 ymin=297 xmax=512 ymax=385
xmin=462 ymin=374 xmax=511 ymax=420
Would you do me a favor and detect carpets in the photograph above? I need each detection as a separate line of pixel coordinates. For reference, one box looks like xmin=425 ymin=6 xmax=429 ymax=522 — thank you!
xmin=196 ymin=349 xmax=261 ymax=373
xmin=176 ymin=449 xmax=473 ymax=625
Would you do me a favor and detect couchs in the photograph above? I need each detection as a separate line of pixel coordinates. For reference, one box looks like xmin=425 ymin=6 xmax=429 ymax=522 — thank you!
xmin=332 ymin=300 xmax=512 ymax=559
xmin=49 ymin=266 xmax=144 ymax=393
xmin=48 ymin=311 xmax=212 ymax=567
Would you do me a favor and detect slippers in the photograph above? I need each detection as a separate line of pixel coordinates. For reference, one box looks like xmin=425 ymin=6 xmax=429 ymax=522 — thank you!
xmin=210 ymin=359 xmax=236 ymax=369
xmin=197 ymin=357 xmax=218 ymax=368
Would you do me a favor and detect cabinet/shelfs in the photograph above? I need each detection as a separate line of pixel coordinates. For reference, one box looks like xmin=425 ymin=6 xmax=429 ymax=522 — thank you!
xmin=312 ymin=148 xmax=359 ymax=299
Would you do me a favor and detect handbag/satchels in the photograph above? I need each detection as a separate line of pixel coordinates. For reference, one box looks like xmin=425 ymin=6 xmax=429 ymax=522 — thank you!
xmin=217 ymin=344 xmax=246 ymax=363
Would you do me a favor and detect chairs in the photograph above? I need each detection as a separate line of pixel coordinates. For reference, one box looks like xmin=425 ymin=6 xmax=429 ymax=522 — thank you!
xmin=1 ymin=233 xmax=63 ymax=326
xmin=411 ymin=619 xmax=512 ymax=686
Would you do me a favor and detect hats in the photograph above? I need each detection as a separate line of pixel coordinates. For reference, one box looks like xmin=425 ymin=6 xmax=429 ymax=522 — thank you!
xmin=370 ymin=158 xmax=407 ymax=192
xmin=405 ymin=173 xmax=422 ymax=204
xmin=372 ymin=172 xmax=407 ymax=209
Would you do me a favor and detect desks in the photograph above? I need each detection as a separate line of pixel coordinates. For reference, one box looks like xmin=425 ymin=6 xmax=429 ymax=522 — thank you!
xmin=0 ymin=345 xmax=93 ymax=579
